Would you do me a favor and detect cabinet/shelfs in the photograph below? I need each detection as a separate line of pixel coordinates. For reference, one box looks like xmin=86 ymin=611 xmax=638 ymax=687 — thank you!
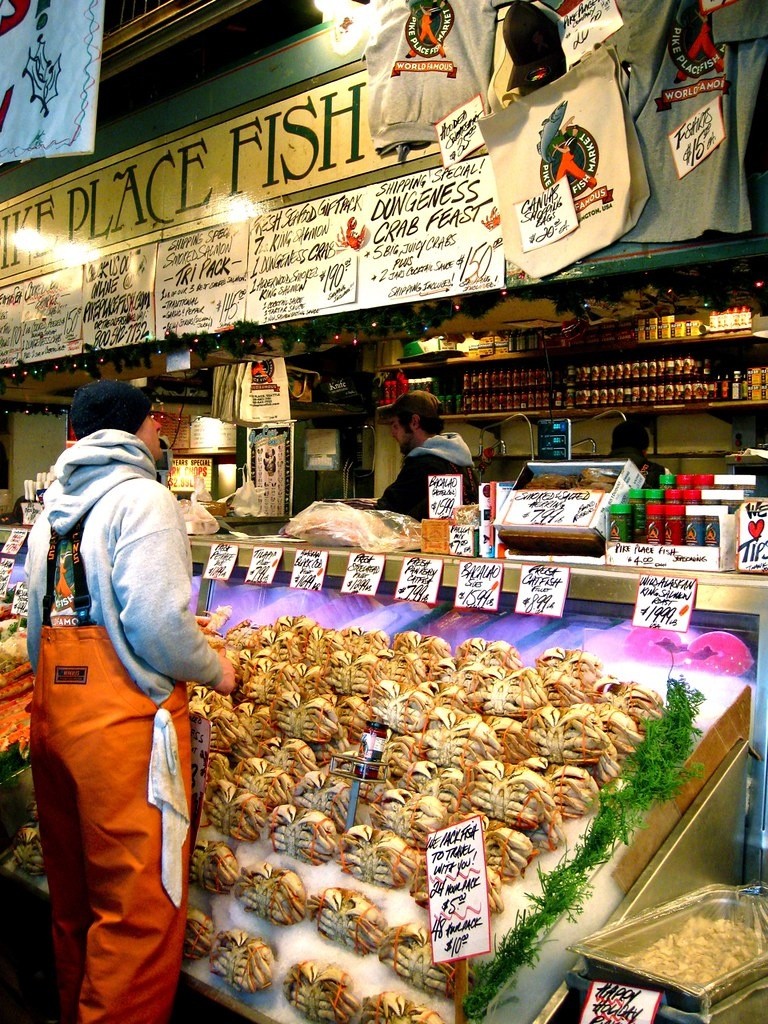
xmin=375 ymin=334 xmax=768 ymax=420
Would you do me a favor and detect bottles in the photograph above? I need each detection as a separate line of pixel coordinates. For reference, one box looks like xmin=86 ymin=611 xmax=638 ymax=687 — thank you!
xmin=610 ymin=474 xmax=757 ymax=548
xmin=377 ymin=366 xmax=747 ymax=414
xmin=354 ymin=721 xmax=389 ymax=780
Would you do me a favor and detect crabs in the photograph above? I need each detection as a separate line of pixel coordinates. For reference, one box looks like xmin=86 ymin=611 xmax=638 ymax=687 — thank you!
xmin=181 ymin=616 xmax=663 ymax=1024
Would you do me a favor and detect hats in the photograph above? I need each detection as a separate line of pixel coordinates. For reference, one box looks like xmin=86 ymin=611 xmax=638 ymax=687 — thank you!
xmin=71 ymin=380 xmax=151 ymax=441
xmin=611 ymin=422 xmax=649 ymax=449
xmin=377 ymin=391 xmax=442 ymax=418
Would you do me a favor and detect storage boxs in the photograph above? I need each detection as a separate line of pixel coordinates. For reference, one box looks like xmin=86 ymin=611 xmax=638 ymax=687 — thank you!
xmin=449 ymin=527 xmax=479 ymax=559
xmin=493 ymin=459 xmax=645 ymax=535
xmin=607 ymin=514 xmax=734 ymax=571
xmin=420 ymin=519 xmax=449 ymax=555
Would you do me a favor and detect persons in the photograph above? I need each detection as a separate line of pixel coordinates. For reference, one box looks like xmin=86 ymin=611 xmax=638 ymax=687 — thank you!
xmin=27 ymin=381 xmax=238 ymax=1024
xmin=610 ymin=422 xmax=664 ymax=488
xmin=362 ymin=390 xmax=479 ymax=522
